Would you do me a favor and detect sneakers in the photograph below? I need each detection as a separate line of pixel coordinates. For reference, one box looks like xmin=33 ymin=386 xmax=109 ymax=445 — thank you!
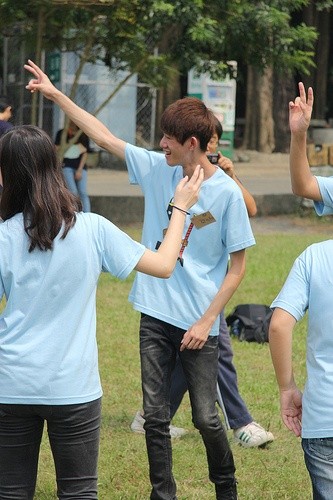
xmin=130 ymin=409 xmax=189 ymax=439
xmin=233 ymin=422 xmax=274 ymax=450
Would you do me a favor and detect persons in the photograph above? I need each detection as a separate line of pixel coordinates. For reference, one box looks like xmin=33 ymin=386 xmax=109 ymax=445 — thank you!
xmin=24 ymin=58 xmax=257 ymax=500
xmin=267 ymin=238 xmax=333 ymax=500
xmin=130 ymin=114 xmax=274 ymax=448
xmin=0 ymin=124 xmax=206 ymax=500
xmin=288 ymin=80 xmax=333 ymax=218
xmin=54 ymin=119 xmax=91 ymax=212
xmin=0 ymin=97 xmax=14 ymax=136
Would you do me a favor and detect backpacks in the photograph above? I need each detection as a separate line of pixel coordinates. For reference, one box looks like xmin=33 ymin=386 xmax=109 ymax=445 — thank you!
xmin=224 ymin=303 xmax=274 ymax=344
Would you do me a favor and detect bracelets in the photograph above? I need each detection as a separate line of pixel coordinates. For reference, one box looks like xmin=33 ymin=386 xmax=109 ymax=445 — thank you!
xmin=168 ymin=202 xmax=191 ymax=216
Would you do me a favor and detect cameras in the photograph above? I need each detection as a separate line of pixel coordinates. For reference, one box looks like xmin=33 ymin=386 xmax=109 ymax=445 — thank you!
xmin=207 ymin=154 xmax=219 ymax=164
